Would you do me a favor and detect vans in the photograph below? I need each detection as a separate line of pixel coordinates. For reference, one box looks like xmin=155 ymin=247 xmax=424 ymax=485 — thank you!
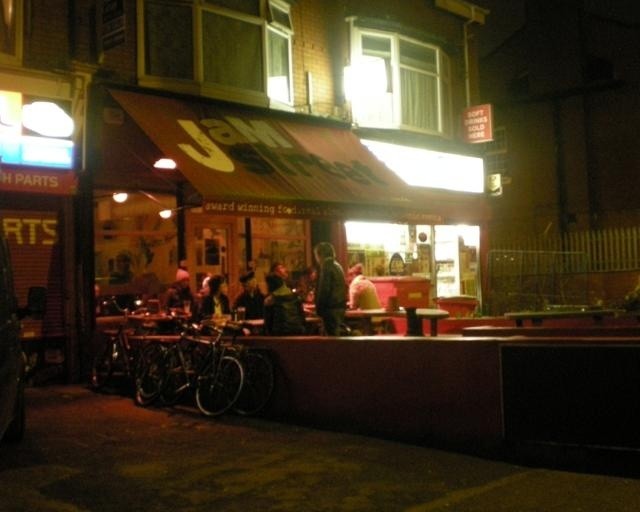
xmin=0 ymin=219 xmax=51 ymax=442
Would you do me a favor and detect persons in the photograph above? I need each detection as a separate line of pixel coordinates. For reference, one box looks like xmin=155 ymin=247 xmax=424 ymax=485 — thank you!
xmin=102 ymin=241 xmax=385 ymax=336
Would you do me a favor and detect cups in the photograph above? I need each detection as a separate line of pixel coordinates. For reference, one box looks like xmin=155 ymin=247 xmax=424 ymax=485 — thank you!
xmin=230 ymin=307 xmax=246 ymax=322
xmin=183 ymin=301 xmax=191 ymax=313
xmin=384 ymin=296 xmax=400 ymax=312
xmin=133 ymin=297 xmax=176 ymax=316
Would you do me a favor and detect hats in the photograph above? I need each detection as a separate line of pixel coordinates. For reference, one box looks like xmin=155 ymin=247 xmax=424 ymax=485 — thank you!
xmin=239 ymin=271 xmax=255 ymax=283
xmin=176 ymin=268 xmax=191 ymax=282
xmin=347 ymin=262 xmax=363 ymax=274
xmin=264 ymin=272 xmax=283 ymax=293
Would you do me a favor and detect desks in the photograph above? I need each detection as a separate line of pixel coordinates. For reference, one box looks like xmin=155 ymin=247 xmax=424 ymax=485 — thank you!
xmin=503 ymin=307 xmax=628 ymax=328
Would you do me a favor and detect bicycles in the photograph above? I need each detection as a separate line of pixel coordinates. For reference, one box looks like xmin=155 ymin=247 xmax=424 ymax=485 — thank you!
xmin=86 ymin=292 xmax=279 ymax=419
